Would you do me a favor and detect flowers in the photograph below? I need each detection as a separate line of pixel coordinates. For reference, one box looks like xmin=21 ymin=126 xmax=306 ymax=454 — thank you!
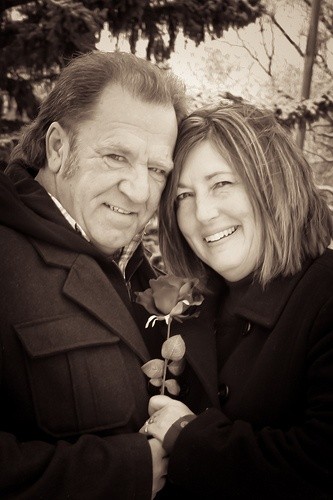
xmin=133 ymin=272 xmax=205 ymax=396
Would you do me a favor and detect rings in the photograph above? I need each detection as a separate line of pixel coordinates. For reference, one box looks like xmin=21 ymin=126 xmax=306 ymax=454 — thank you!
xmin=146 ymin=417 xmax=153 ymax=424
xmin=144 ymin=424 xmax=150 ymax=436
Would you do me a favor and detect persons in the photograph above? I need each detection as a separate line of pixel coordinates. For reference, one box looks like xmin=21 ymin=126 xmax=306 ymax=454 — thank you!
xmin=138 ymin=101 xmax=333 ymax=500
xmin=0 ymin=52 xmax=184 ymax=500
